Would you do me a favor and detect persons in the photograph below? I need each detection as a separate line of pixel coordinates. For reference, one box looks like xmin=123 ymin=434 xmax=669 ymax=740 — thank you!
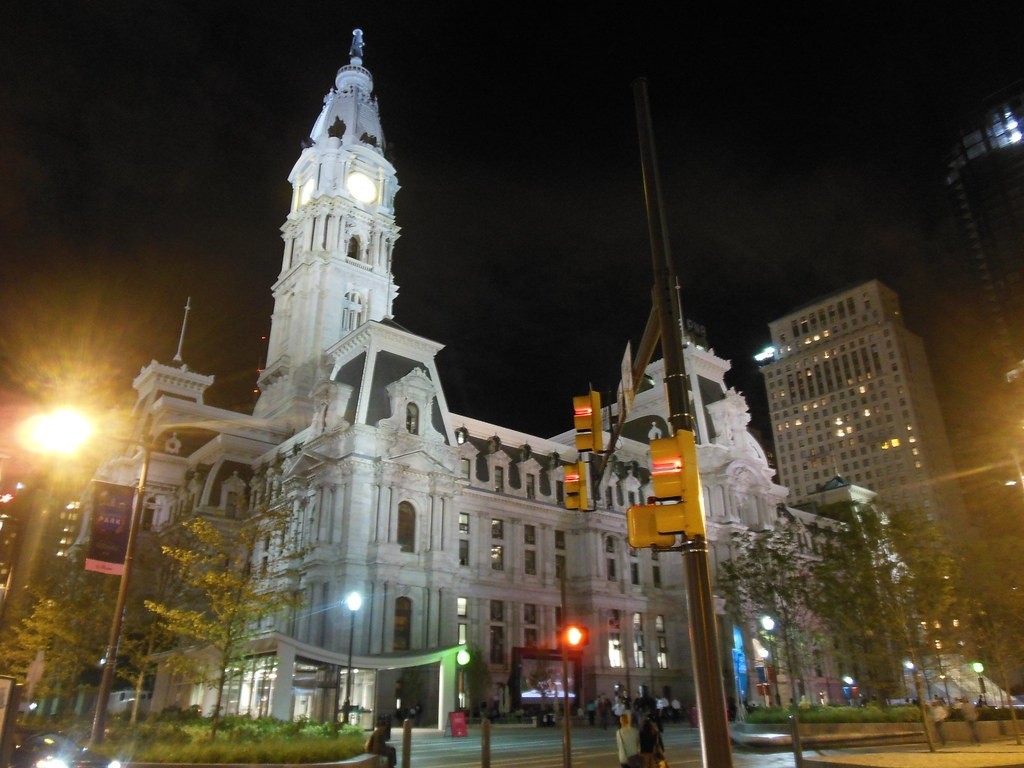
xmin=930 ymin=695 xmax=976 ymax=747
xmin=979 ymin=694 xmax=983 ymax=705
xmin=904 ymin=698 xmax=917 ymax=705
xmin=617 ymin=714 xmax=665 ymax=768
xmin=859 ymin=695 xmax=891 ymax=707
xmin=788 ymin=695 xmax=808 ymax=712
xmin=587 ymin=693 xmax=681 ymax=731
xmin=371 ymin=722 xmax=397 ymax=768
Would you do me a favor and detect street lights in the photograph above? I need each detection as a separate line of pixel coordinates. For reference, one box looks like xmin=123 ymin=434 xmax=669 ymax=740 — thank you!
xmin=760 ymin=615 xmax=782 ymax=707
xmin=14 ymin=405 xmax=160 ymax=744
xmin=343 ymin=589 xmax=364 ymax=725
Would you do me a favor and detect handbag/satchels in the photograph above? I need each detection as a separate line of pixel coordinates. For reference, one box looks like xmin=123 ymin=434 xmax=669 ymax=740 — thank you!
xmin=627 ymin=754 xmax=645 ymax=768
xmin=648 ymin=757 xmax=667 ymax=768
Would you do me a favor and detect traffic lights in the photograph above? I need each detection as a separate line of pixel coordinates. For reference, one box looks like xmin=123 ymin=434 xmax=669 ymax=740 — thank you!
xmin=572 ymin=391 xmax=604 ymax=454
xmin=561 ymin=623 xmax=590 ymax=650
xmin=563 ymin=460 xmax=589 ymax=512
xmin=649 ymin=430 xmax=706 ymax=538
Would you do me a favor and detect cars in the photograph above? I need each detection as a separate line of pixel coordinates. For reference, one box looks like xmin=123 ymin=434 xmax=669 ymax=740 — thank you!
xmin=9 ymin=731 xmax=122 ymax=768
